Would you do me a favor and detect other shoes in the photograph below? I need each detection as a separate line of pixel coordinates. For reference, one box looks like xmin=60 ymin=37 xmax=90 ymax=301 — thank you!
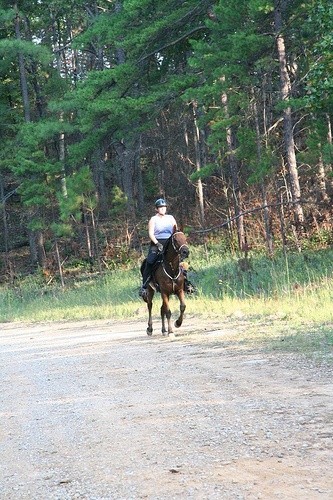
xmin=139 ymin=286 xmax=148 ymax=297
xmin=185 ymin=282 xmax=195 ymax=294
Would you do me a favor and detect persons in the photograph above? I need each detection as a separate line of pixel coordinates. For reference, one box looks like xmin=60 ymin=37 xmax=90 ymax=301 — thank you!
xmin=141 ymin=198 xmax=196 ymax=303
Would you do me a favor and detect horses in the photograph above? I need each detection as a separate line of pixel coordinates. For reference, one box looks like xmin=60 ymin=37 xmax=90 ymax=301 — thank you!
xmin=147 ymin=224 xmax=189 ymax=338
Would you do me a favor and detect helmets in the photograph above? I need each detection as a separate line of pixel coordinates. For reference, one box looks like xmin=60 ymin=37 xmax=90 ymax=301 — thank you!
xmin=155 ymin=198 xmax=168 ymax=208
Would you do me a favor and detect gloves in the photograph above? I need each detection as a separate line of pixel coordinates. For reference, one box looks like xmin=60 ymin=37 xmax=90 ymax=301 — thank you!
xmin=156 ymin=242 xmax=163 ymax=251
